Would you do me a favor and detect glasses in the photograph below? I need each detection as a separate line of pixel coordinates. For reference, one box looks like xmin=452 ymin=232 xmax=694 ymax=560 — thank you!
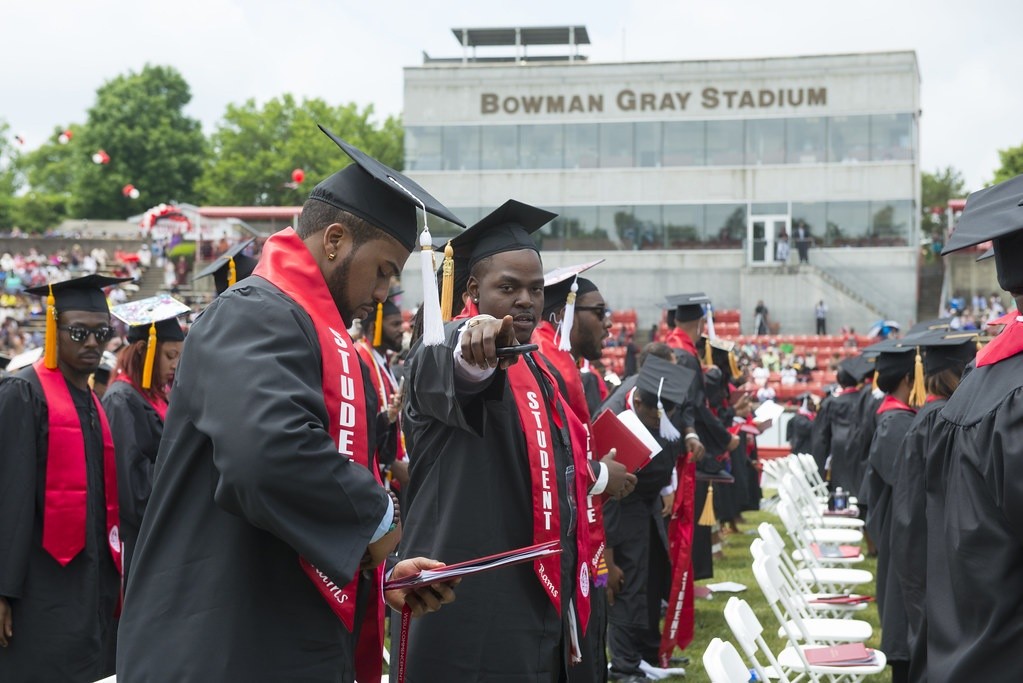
xmin=574 ymin=303 xmax=606 ymax=321
xmin=59 ymin=325 xmax=116 ymax=344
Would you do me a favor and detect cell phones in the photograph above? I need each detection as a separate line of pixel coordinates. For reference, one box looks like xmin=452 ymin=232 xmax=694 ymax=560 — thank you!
xmin=461 ymin=344 xmax=540 ymax=359
xmin=394 ymin=377 xmax=405 ymax=407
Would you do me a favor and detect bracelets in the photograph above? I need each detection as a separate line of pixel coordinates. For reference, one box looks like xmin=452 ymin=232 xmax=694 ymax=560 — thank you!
xmin=386 ymin=488 xmax=401 ymax=536
xmin=685 ymin=433 xmax=699 ymax=441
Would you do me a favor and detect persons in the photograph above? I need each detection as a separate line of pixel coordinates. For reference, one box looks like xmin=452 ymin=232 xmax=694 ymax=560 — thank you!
xmin=796 ymin=223 xmax=810 ymax=263
xmin=0 ymin=222 xmax=255 ymax=362
xmin=102 ymin=293 xmax=191 ymax=595
xmin=735 ymin=338 xmax=817 ymax=402
xmin=389 ymin=198 xmax=763 ymax=683
xmin=790 ymin=173 xmax=1023 ymax=683
xmin=776 ymin=234 xmax=789 ymax=262
xmin=115 ymin=125 xmax=467 ymax=683
xmin=353 ymin=290 xmax=409 ymax=487
xmin=0 ymin=274 xmax=125 ymax=683
xmin=816 ymin=299 xmax=827 ymax=335
xmin=755 ymin=301 xmax=769 ymax=335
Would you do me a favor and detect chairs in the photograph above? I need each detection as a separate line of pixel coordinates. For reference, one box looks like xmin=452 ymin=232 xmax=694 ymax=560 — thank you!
xmin=701 ymin=452 xmax=889 ymax=683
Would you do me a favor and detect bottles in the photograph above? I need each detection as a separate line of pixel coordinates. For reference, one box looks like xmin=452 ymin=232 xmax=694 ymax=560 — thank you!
xmin=834 ymin=487 xmax=845 ymax=512
xmin=748 ymin=665 xmax=780 ymax=682
xmin=744 ymin=528 xmax=758 ymax=535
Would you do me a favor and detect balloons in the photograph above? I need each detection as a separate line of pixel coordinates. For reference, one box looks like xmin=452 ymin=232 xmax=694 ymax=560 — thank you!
xmin=92 ymin=149 xmax=110 ymax=165
xmin=122 ymin=184 xmax=140 ymax=199
xmin=59 ymin=130 xmax=72 ymax=144
xmin=293 ymin=169 xmax=304 ymax=183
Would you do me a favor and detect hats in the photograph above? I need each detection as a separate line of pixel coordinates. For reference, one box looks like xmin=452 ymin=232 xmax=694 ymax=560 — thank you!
xmin=435 ymin=198 xmax=560 ymax=324
xmin=696 ymin=453 xmax=725 ymax=527
xmin=862 ymin=351 xmax=880 ymax=391
xmin=860 ymin=338 xmax=927 ymax=407
xmin=902 ymin=329 xmax=982 ymax=358
xmin=110 ymin=293 xmax=192 ymax=389
xmin=634 ymin=353 xmax=698 ymax=442
xmin=360 ymin=287 xmax=403 ymax=346
xmin=193 ymin=237 xmax=260 ymax=286
xmin=654 ymin=292 xmax=747 ymax=381
xmin=22 ymin=273 xmax=135 ymax=369
xmin=839 ymin=356 xmax=875 ymax=390
xmin=544 ymin=258 xmax=607 ymax=352
xmin=308 ymin=122 xmax=467 ymax=347
xmin=941 ymin=174 xmax=1023 ymax=256
xmin=796 ymin=391 xmax=809 ymax=409
